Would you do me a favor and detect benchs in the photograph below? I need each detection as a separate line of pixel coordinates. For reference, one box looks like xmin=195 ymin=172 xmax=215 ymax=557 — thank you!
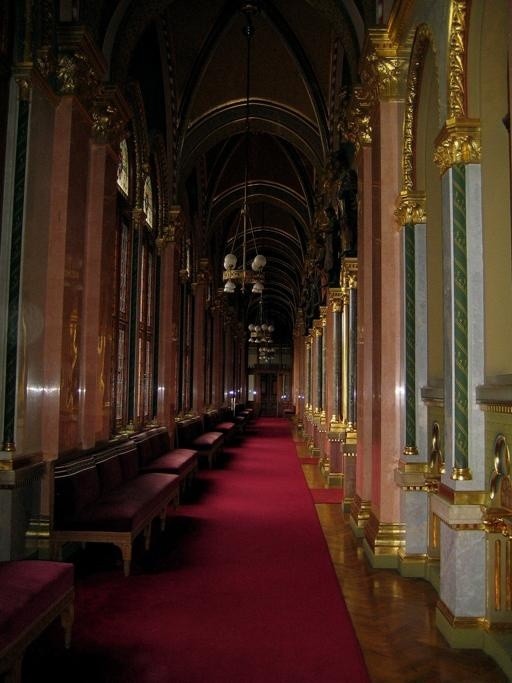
xmin=50 ymin=440 xmax=179 ymax=577
xmin=1 ymin=401 xmax=256 ymax=683
xmin=132 ymin=427 xmax=197 ymax=503
xmin=173 ymin=403 xmax=255 ymax=468
xmin=2 ymin=560 xmax=73 ymax=681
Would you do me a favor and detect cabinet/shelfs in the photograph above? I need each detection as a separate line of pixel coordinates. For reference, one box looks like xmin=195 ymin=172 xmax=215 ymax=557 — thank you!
xmin=1 ymin=451 xmax=45 ymax=562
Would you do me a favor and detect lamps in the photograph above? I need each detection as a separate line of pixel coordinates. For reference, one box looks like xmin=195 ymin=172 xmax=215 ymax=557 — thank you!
xmin=222 ymin=0 xmax=265 ymax=295
xmin=248 ymin=295 xmax=274 ymax=344
xmin=258 ymin=344 xmax=277 ymax=363
